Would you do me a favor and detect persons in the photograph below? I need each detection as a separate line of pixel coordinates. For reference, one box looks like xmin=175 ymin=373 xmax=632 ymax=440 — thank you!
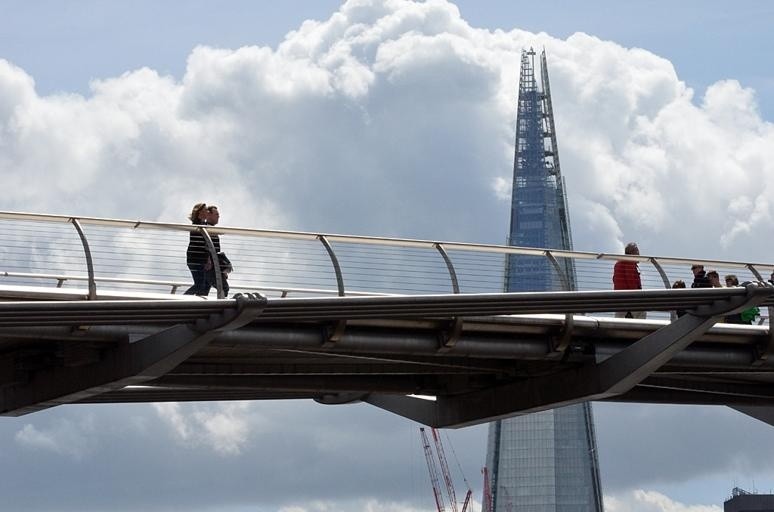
xmin=184 ymin=204 xmax=211 ymax=295
xmin=673 ymin=265 xmax=774 ymax=326
xmin=206 ymin=207 xmax=230 ymax=298
xmin=612 ymin=242 xmax=646 ymax=320
